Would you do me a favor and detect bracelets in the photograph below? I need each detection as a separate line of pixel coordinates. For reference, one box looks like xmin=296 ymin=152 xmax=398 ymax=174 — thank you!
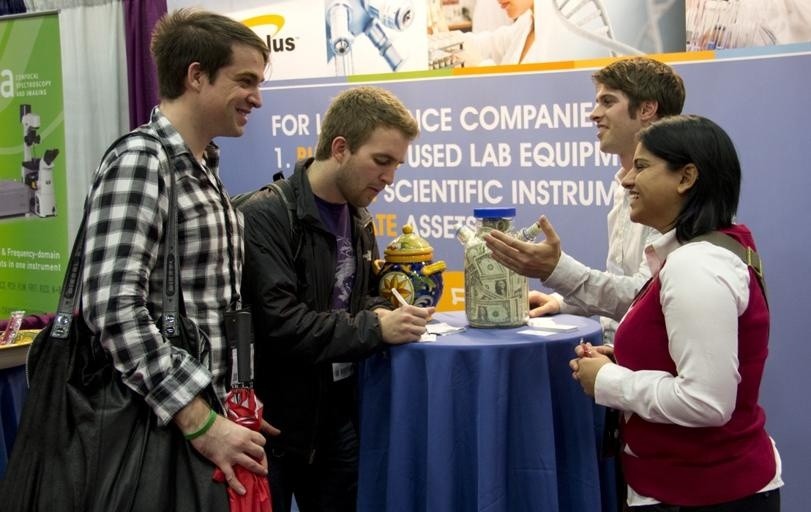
xmin=182 ymin=406 xmax=221 ymax=442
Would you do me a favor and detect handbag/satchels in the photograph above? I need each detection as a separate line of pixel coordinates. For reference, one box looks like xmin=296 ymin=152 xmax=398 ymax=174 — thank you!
xmin=0 ymin=312 xmax=233 ymax=512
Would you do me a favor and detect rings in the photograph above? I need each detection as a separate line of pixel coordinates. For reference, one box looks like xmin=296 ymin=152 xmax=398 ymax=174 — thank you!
xmin=509 ymin=257 xmax=514 ymax=265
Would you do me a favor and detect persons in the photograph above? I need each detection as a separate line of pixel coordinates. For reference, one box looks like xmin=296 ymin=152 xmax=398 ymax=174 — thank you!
xmin=495 ymin=0 xmax=544 ymax=64
xmin=80 ymin=8 xmax=284 ymax=511
xmin=480 ymin=56 xmax=686 ymax=348
xmin=226 ymin=85 xmax=438 ymax=510
xmin=570 ymin=112 xmax=783 ymax=508
xmin=696 ymin=2 xmax=811 ymax=51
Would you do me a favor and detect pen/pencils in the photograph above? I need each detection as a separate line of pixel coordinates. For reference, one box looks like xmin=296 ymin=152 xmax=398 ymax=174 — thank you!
xmin=391 ymin=288 xmax=429 ymax=335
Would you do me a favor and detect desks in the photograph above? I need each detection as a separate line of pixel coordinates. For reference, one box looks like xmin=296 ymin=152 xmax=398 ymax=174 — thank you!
xmin=358 ymin=310 xmax=623 ymax=512
